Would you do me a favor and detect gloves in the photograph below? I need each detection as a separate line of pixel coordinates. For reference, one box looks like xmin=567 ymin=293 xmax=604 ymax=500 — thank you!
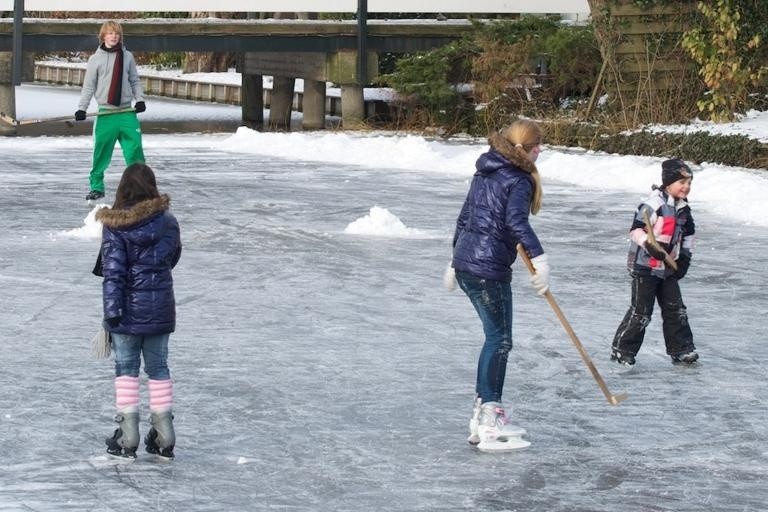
xmin=75 ymin=110 xmax=86 ymax=121
xmin=530 ymin=253 xmax=550 ymax=295
xmin=674 ymin=254 xmax=690 ymax=278
xmin=645 ymin=241 xmax=665 ymax=260
xmin=135 ymin=102 xmax=145 ymax=112
xmin=445 ymin=260 xmax=456 ymax=292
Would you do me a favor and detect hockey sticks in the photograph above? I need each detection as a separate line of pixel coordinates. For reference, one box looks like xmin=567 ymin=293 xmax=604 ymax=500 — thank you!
xmin=643 ymin=208 xmax=681 ymax=272
xmin=517 ymin=243 xmax=630 ymax=407
xmin=1 ymin=107 xmax=137 ymax=126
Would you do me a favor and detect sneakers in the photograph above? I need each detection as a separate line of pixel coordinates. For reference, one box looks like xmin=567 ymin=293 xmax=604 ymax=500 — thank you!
xmin=86 ymin=190 xmax=104 ymax=199
xmin=613 ymin=350 xmax=635 ymax=364
xmin=671 ymin=352 xmax=698 ymax=362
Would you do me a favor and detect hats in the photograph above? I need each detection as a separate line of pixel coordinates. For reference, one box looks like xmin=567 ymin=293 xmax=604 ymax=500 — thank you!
xmin=662 ymin=159 xmax=692 ymax=186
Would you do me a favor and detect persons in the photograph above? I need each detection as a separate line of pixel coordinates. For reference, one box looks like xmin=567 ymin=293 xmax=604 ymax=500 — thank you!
xmin=72 ymin=19 xmax=148 ymax=200
xmin=91 ymin=163 xmax=183 ymax=455
xmin=444 ymin=118 xmax=554 ymax=444
xmin=609 ymin=157 xmax=702 ymax=369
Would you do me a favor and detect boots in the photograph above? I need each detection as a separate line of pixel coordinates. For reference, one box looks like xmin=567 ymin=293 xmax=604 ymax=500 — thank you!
xmin=144 ymin=412 xmax=175 ymax=448
xmin=471 ymin=398 xmax=526 ymax=437
xmin=105 ymin=412 xmax=140 ymax=449
